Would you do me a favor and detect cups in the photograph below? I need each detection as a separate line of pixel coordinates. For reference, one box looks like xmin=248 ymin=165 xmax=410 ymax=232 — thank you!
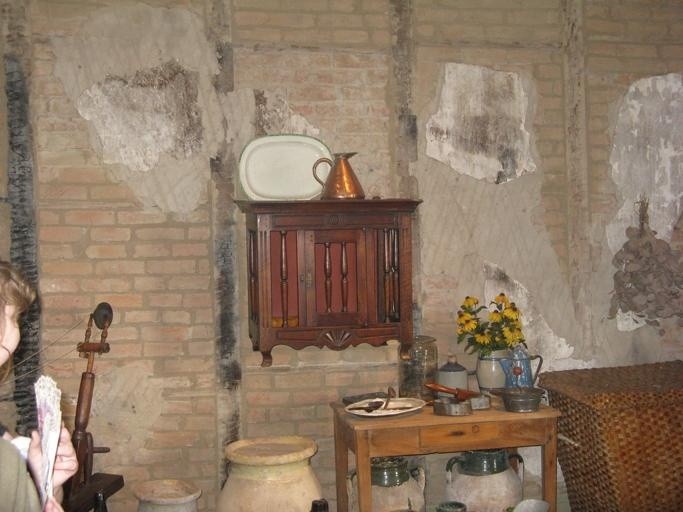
xmin=436 ymin=354 xmax=469 ymax=399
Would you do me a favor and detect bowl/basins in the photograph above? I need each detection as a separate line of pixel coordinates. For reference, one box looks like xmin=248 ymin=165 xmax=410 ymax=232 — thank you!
xmin=501 ymin=386 xmax=545 ymax=413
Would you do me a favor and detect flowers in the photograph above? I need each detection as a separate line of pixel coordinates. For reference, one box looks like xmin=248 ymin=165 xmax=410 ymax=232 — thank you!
xmin=455 ymin=291 xmax=529 ymax=356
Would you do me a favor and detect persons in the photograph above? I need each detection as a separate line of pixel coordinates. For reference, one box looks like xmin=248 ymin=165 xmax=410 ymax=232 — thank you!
xmin=0 ymin=260 xmax=79 ymax=512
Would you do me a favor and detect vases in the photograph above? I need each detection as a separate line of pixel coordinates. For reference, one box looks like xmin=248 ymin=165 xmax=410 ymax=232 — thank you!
xmin=476 ymin=349 xmax=512 ymax=397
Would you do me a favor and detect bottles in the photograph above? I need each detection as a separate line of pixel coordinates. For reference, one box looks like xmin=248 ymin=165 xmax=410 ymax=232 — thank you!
xmin=409 ymin=336 xmax=438 ymax=401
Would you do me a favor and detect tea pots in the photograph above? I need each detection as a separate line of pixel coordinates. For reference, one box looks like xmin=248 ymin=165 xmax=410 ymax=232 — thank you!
xmin=500 ymin=343 xmax=543 ymax=387
xmin=313 ymin=152 xmax=365 ymax=199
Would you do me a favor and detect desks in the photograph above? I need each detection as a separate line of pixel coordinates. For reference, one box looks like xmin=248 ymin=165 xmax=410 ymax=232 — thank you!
xmin=329 ymin=392 xmax=561 ymax=512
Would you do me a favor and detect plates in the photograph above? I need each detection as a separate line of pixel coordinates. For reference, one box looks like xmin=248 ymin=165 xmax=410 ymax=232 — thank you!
xmin=345 ymin=396 xmax=425 ymax=416
xmin=240 ymin=135 xmax=334 ymax=203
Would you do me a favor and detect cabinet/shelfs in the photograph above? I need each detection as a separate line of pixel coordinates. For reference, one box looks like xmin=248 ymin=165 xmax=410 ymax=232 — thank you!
xmin=235 ymin=197 xmax=425 ymax=367
